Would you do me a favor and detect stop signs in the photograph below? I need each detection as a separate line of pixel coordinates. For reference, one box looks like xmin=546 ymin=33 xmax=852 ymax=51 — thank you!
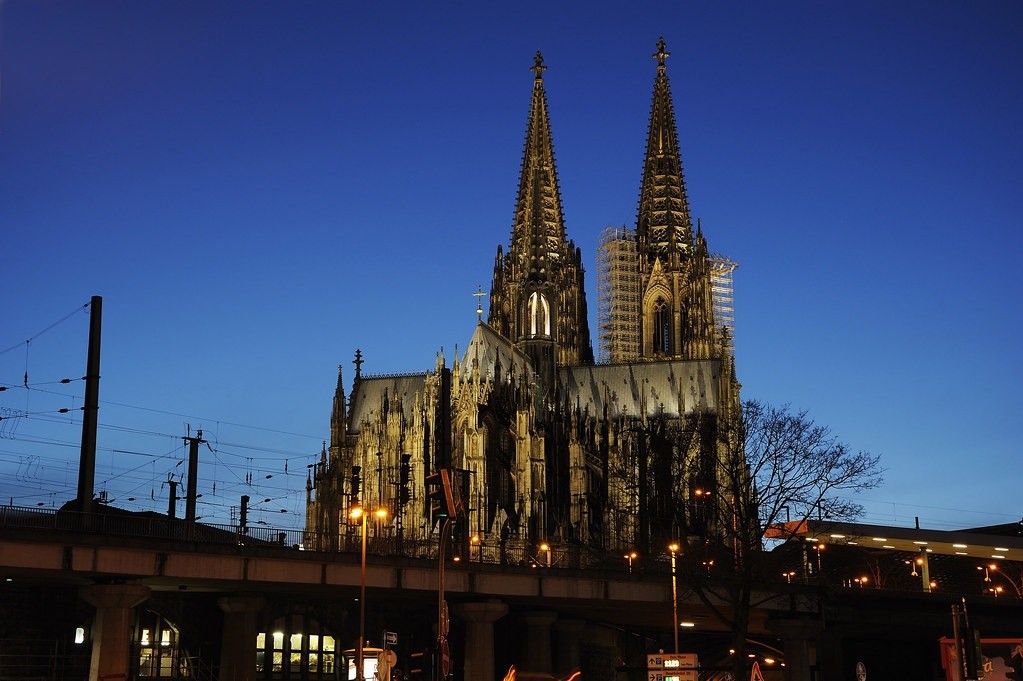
xmin=442 ymin=641 xmax=450 ymax=677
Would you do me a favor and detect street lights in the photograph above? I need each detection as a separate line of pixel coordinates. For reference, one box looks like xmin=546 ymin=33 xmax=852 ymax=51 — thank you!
xmin=349 ymin=505 xmax=388 ymax=681
xmin=780 ymin=506 xmax=790 ymax=523
xmin=540 ymin=544 xmax=552 ymax=568
xmin=624 ymin=553 xmax=637 ymax=574
xmin=978 ymin=565 xmax=997 ymax=582
xmin=667 ymin=542 xmax=680 ymax=656
xmin=854 ymin=577 xmax=867 ymax=589
xmin=783 ymin=571 xmax=795 ymax=584
xmin=812 ymin=544 xmax=824 ymax=573
xmin=905 ymin=558 xmax=923 ymax=575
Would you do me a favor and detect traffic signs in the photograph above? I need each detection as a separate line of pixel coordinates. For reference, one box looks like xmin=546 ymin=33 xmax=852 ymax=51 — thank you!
xmin=647 ymin=653 xmax=699 ymax=670
xmin=647 ymin=670 xmax=699 ymax=681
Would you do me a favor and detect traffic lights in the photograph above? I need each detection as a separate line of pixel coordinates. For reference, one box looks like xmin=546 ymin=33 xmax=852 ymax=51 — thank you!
xmin=425 ymin=468 xmax=457 ymax=521
